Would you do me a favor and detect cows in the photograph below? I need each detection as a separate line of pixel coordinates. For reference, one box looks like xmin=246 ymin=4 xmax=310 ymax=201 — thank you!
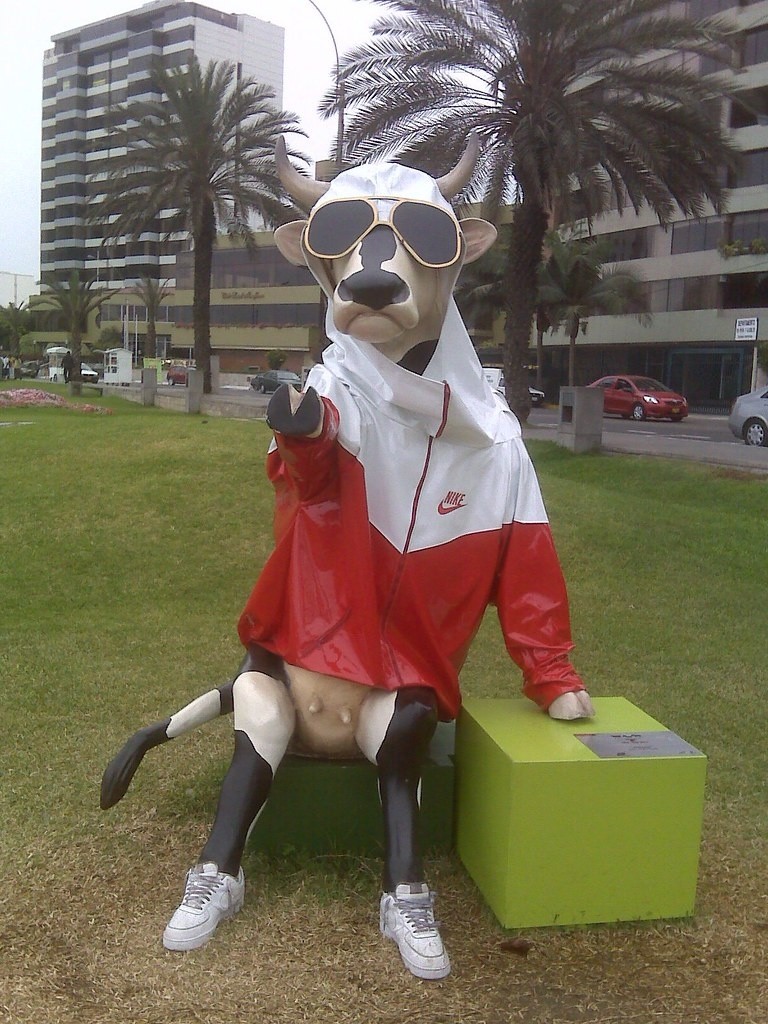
xmin=98 ymin=129 xmax=598 ymax=980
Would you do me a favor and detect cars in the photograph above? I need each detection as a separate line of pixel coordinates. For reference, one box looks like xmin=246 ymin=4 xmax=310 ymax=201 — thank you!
xmin=728 ymin=384 xmax=768 ymax=449
xmin=80 ymin=362 xmax=99 ymax=384
xmin=251 ymin=369 xmax=304 ymax=394
xmin=585 ymin=374 xmax=688 ymax=422
xmin=21 ymin=360 xmax=39 ymax=378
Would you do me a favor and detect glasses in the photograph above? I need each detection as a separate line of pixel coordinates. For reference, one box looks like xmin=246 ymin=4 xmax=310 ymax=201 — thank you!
xmin=303 ymin=194 xmax=463 ymax=271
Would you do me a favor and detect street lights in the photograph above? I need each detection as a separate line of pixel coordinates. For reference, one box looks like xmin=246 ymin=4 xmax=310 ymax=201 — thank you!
xmin=97 ymin=233 xmax=125 ymax=299
xmin=33 ymin=340 xmax=36 ymax=359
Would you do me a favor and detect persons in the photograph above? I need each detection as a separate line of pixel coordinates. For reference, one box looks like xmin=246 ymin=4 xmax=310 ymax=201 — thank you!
xmin=0 ymin=355 xmax=22 ymax=380
xmin=61 ymin=351 xmax=75 ymax=383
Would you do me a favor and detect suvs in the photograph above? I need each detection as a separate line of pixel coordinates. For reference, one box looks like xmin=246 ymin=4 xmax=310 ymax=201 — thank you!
xmin=166 ymin=364 xmax=197 ymax=387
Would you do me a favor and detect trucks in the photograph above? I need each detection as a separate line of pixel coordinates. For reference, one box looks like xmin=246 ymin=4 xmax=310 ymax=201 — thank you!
xmin=481 ymin=366 xmax=545 ymax=408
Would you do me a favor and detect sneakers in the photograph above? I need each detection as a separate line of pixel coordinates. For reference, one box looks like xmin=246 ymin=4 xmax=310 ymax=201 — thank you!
xmin=162 ymin=862 xmax=245 ymax=951
xmin=379 ymin=881 xmax=452 ymax=979
